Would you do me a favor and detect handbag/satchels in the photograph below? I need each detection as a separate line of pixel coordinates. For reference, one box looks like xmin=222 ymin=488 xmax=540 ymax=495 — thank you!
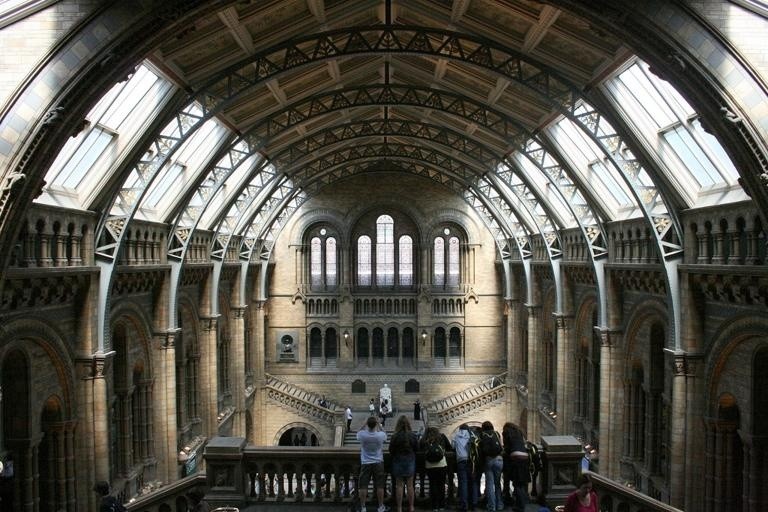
xmin=467 ymin=431 xmax=481 ymax=463
xmin=526 ymin=440 xmax=540 ymax=476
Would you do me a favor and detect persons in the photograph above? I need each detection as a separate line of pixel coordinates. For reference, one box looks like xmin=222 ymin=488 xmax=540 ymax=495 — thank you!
xmin=345 ymin=405 xmax=353 ymax=431
xmin=566 ymin=474 xmax=600 ymax=512
xmin=420 ymin=427 xmax=454 ymax=512
xmin=449 ymin=424 xmax=481 ymax=512
xmin=368 ymin=398 xmax=375 ymax=417
xmin=413 ymin=401 xmax=420 ymax=420
xmin=503 ymin=421 xmax=531 ymax=511
xmin=356 ymin=417 xmax=387 ymax=511
xmin=388 ymin=415 xmax=420 ymax=512
xmin=282 ymin=340 xmax=293 ymax=352
xmin=479 ymin=421 xmax=505 ymax=511
xmin=318 ymin=394 xmax=328 ymax=409
xmin=379 ymin=383 xmax=392 ymax=413
xmin=380 ymin=402 xmax=389 ymax=428
xmin=384 ymin=399 xmax=389 ymax=410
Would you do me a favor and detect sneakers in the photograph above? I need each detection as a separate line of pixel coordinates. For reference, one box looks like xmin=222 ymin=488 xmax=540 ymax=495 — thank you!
xmin=356 ymin=506 xmax=366 ymax=512
xmin=378 ymin=504 xmax=390 ymax=512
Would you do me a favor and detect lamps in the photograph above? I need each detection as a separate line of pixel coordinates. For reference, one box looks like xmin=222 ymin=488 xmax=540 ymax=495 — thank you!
xmin=343 ymin=328 xmax=349 ymax=346
xmin=421 ymin=329 xmax=428 ymax=346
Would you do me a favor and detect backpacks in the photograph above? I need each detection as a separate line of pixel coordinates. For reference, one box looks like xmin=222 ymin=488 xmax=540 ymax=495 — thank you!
xmin=481 ymin=431 xmax=501 ymax=454
xmin=426 ymin=435 xmax=443 ymax=463
